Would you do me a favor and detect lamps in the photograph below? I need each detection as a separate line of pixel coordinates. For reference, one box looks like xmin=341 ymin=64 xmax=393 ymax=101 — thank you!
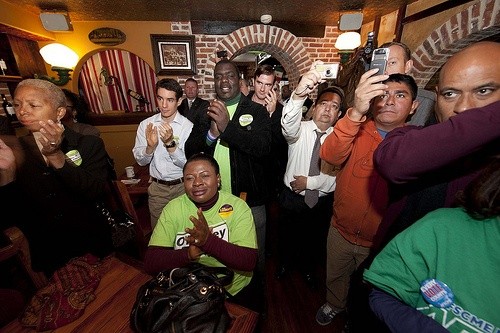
xmin=34 ymin=43 xmax=80 ymax=86
xmin=334 ymin=32 xmax=362 ymax=65
xmin=338 ymin=6 xmax=363 ymax=31
xmin=40 ymin=6 xmax=74 ymax=32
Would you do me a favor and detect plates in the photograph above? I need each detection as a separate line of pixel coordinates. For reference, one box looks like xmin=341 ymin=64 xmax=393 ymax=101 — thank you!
xmin=121 ymin=179 xmax=141 ymax=184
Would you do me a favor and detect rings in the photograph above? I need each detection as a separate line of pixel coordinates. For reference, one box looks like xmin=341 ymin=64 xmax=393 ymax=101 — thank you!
xmin=193 ymin=239 xmax=198 ymax=244
xmin=50 ymin=141 xmax=56 ymax=145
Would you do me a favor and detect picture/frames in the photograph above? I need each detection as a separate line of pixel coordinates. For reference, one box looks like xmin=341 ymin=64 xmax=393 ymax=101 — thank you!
xmin=150 ymin=34 xmax=197 ymax=76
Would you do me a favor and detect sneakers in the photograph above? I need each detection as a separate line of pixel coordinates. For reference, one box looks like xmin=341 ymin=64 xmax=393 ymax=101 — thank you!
xmin=316 ymin=303 xmax=339 ymax=326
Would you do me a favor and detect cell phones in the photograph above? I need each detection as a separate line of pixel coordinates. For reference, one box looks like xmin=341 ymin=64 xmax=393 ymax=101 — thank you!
xmin=369 ymin=48 xmax=389 ymax=84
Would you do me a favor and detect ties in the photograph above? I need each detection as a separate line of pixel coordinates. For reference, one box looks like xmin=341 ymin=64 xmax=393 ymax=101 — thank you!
xmin=305 ymin=132 xmax=326 ymax=209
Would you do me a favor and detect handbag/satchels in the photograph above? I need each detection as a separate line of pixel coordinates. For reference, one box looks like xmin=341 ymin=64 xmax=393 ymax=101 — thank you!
xmin=130 ymin=263 xmax=234 ymax=333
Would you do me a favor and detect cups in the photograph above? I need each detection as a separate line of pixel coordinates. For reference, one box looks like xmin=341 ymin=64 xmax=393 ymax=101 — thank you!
xmin=125 ymin=166 xmax=135 ymax=177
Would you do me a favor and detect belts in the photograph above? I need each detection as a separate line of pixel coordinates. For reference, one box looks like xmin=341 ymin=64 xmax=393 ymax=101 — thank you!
xmin=152 ymin=177 xmax=183 ymax=185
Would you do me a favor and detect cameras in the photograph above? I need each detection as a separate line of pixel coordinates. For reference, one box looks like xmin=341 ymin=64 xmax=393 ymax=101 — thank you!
xmin=315 ymin=62 xmax=339 ymax=79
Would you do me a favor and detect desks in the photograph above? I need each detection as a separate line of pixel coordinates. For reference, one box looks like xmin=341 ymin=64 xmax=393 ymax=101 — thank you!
xmin=0 ymin=253 xmax=259 ymax=333
xmin=119 ymin=161 xmax=152 ymax=193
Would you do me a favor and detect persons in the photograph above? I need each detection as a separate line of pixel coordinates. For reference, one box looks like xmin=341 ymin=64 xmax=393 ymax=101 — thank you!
xmin=316 ymin=69 xmax=420 ymax=327
xmin=176 ymin=78 xmax=313 ymax=158
xmin=133 ymin=79 xmax=195 ymax=233
xmin=343 ymin=41 xmax=500 ymax=333
xmin=61 ymin=89 xmax=100 ymax=136
xmin=274 ymin=61 xmax=343 ymax=286
xmin=144 ymin=155 xmax=267 ymax=333
xmin=246 ymin=64 xmax=288 ymax=279
xmin=185 ymin=60 xmax=272 ymax=270
xmin=0 ymin=80 xmax=138 ymax=278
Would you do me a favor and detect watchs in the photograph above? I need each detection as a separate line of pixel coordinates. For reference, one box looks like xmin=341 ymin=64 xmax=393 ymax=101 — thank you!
xmin=165 ymin=141 xmax=175 ymax=148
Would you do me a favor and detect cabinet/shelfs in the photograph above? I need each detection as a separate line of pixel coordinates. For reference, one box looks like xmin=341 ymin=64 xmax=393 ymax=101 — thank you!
xmin=0 ymin=75 xmax=23 ymax=123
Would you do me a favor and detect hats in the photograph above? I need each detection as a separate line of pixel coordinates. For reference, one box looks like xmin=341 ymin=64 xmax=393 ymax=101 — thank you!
xmin=321 ymin=85 xmax=346 ymax=104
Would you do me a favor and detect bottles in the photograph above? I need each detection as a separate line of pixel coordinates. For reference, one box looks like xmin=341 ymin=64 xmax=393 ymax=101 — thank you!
xmin=1 ymin=93 xmax=18 ymax=121
xmin=127 ymin=89 xmax=151 ymax=104
